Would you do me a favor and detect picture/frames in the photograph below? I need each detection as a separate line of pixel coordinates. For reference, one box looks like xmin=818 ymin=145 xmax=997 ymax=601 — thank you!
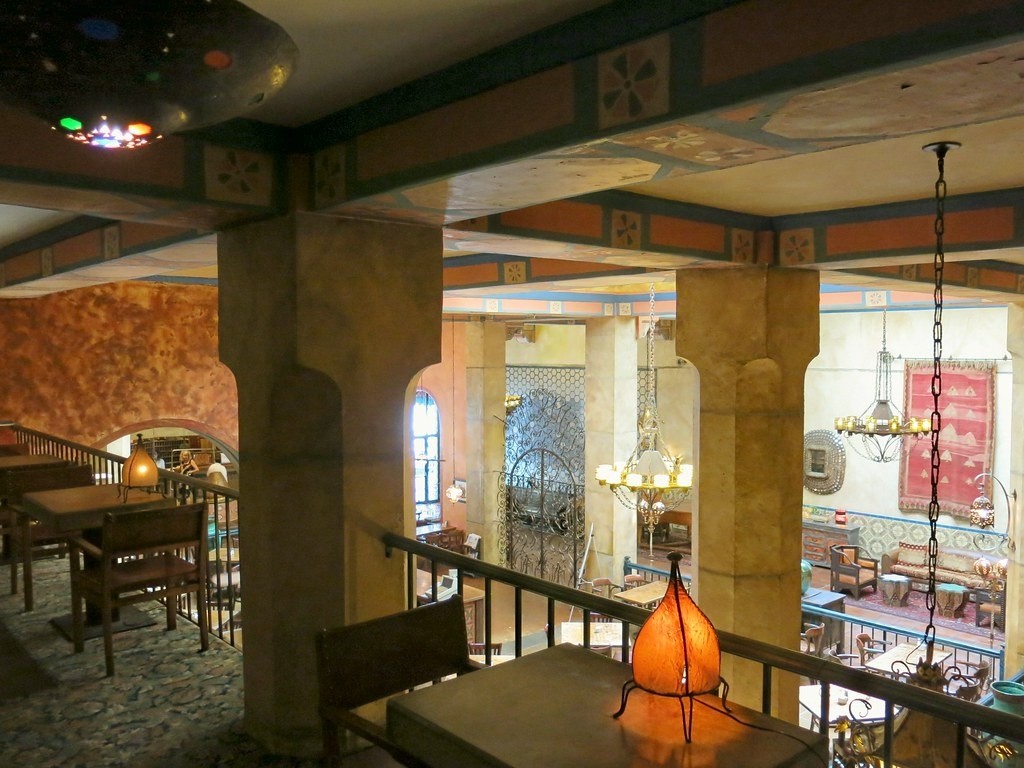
xmin=453 ymin=478 xmax=467 ymax=503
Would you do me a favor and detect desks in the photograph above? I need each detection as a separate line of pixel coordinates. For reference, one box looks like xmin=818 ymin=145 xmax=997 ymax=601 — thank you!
xmin=934 ymin=583 xmax=970 ymax=618
xmin=210 ymin=548 xmax=240 ymax=572
xmin=799 ymin=684 xmax=899 ymax=739
xmin=385 ymin=644 xmax=829 ymax=768
xmin=614 ymin=580 xmax=691 ymax=609
xmin=879 ymin=573 xmax=911 ymax=607
xmin=417 ymin=523 xmax=456 ymax=546
xmin=0 ymin=453 xmax=70 ymax=595
xmin=561 ymin=621 xmax=632 ymax=648
xmin=181 ymin=608 xmax=240 ymax=631
xmin=19 ymin=483 xmax=177 ymax=652
xmin=222 ymin=629 xmax=243 ymax=648
xmin=866 ymin=642 xmax=953 ymax=683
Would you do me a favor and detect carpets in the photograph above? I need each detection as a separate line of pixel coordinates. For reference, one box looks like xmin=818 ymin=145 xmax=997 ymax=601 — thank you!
xmin=844 ymin=580 xmax=1006 ymax=642
xmin=638 ymin=547 xmax=692 ymax=567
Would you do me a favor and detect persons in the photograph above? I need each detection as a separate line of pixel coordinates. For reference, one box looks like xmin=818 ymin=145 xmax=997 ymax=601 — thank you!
xmin=179 ymin=451 xmax=199 ymax=476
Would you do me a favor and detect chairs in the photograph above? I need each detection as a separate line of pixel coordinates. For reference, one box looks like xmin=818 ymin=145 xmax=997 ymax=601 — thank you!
xmin=944 ymin=679 xmax=980 ymax=701
xmin=469 ymin=642 xmax=502 ymax=656
xmin=975 ymin=581 xmax=1006 ymax=633
xmin=828 ymin=649 xmax=866 ymax=670
xmin=0 ymin=443 xmax=31 ymax=457
xmin=314 ymin=594 xmax=491 ymax=768
xmin=801 ymin=622 xmax=825 ymax=686
xmin=855 ymin=633 xmax=891 ymax=675
xmin=418 ymin=534 xmax=444 ymax=547
xmin=590 ymin=613 xmax=613 ymax=623
xmin=578 ymin=644 xmax=617 ymax=660
xmin=830 ymin=545 xmax=880 ymax=600
xmin=69 ymin=500 xmax=210 ymax=677
xmin=7 ymin=464 xmax=96 ymax=611
xmin=832 ymin=715 xmax=886 ymax=768
xmin=624 ymin=574 xmax=653 ymax=611
xmin=591 ymin=578 xmax=623 ymax=601
xmin=950 ymin=660 xmax=991 ymax=700
xmin=441 ymin=529 xmax=464 ymax=554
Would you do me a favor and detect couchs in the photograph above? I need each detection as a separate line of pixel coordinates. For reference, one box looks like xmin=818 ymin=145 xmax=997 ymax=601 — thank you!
xmin=882 ymin=541 xmax=1008 ymax=603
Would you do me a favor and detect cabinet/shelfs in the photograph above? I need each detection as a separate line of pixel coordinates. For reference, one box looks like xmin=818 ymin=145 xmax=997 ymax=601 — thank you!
xmin=802 ymin=518 xmax=861 ymax=568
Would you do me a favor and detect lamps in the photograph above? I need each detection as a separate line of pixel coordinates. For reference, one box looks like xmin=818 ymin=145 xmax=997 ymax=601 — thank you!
xmin=973 ymin=555 xmax=1009 ymax=650
xmin=505 ymin=394 xmax=524 ymax=417
xmin=612 ymin=550 xmax=732 ymax=745
xmin=446 ymin=485 xmax=465 ymax=503
xmin=595 ymin=283 xmax=694 ymax=534
xmin=834 ymin=309 xmax=933 ymax=463
xmin=829 ymin=139 xmax=1024 ymax=768
xmin=969 ymin=473 xmax=1017 ymax=553
xmin=116 ymin=433 xmax=166 ymax=503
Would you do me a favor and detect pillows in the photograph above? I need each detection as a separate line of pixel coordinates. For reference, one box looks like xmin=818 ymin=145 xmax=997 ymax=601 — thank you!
xmin=897 ymin=541 xmax=928 ymax=568
